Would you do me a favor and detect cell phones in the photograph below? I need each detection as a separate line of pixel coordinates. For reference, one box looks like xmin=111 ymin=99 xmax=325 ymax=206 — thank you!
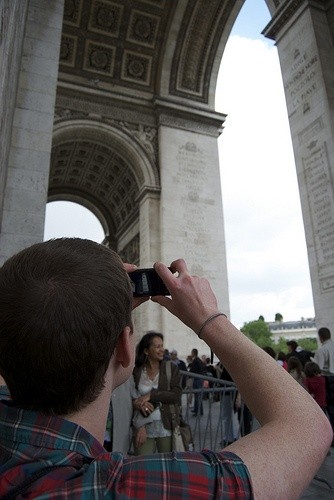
xmin=128 ymin=267 xmax=172 ymax=297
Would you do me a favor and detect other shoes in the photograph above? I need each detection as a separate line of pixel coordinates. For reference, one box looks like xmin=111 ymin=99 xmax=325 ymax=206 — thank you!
xmin=191 ymin=410 xmax=204 ymax=416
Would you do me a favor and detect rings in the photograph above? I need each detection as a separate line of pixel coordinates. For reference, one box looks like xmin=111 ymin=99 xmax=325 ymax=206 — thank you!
xmin=145 ymin=408 xmax=148 ymax=410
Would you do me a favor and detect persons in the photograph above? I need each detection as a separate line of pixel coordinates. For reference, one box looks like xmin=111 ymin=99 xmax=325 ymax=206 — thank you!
xmin=104 ymin=330 xmax=183 ymax=456
xmin=261 ymin=325 xmax=334 ymax=419
xmin=170 ymin=349 xmax=254 ymax=448
xmin=0 ymin=237 xmax=334 ymax=500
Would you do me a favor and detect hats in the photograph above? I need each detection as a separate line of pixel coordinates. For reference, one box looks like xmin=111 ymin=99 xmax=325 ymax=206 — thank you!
xmin=285 ymin=340 xmax=298 ymax=348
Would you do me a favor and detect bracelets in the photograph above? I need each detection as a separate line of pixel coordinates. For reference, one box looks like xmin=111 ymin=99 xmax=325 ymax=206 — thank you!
xmin=198 ymin=313 xmax=227 ymax=339
